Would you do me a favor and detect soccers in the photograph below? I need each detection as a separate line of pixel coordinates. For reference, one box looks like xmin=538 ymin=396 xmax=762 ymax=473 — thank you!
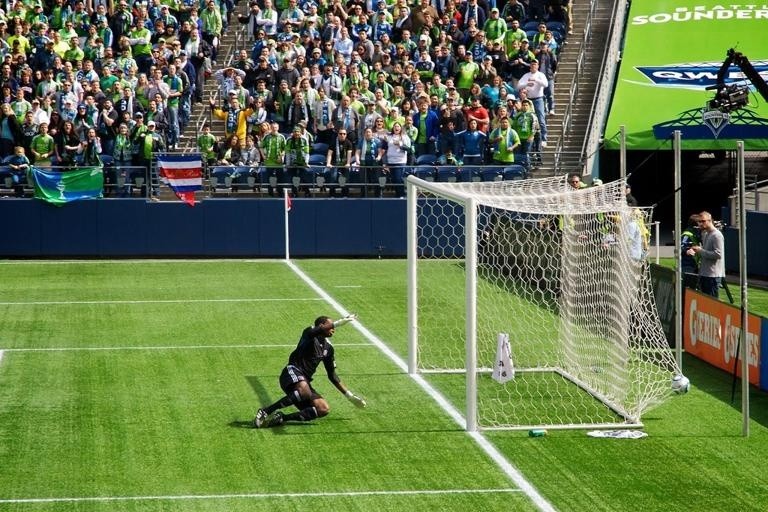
xmin=671 ymin=375 xmax=691 ymax=395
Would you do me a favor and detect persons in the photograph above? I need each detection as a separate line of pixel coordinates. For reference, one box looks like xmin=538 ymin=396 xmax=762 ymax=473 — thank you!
xmin=254 ymin=312 xmax=366 ymax=427
xmin=589 ymin=179 xmax=612 ymax=239
xmin=626 ymin=184 xmax=637 ymax=207
xmin=1 ymin=0 xmax=573 ymax=198
xmin=681 ymin=214 xmax=703 ymax=291
xmin=692 ymin=212 xmax=725 ymax=300
xmin=558 ymin=175 xmax=587 ymax=238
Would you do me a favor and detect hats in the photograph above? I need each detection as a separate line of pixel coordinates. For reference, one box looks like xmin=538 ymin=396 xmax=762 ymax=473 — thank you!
xmin=594 ymin=179 xmax=603 ymax=186
xmin=378 ymin=0 xmax=387 ymax=15
xmin=283 ymin=18 xmax=321 ymax=62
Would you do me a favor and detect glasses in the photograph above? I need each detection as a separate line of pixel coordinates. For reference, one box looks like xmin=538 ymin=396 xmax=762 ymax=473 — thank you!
xmin=699 ymin=220 xmax=709 ymax=223
xmin=572 ymin=181 xmax=578 ymax=183
xmin=339 ymin=133 xmax=346 ymax=135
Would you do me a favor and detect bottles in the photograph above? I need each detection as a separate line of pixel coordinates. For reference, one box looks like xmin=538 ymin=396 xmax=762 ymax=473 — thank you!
xmin=529 ymin=430 xmax=548 ymax=437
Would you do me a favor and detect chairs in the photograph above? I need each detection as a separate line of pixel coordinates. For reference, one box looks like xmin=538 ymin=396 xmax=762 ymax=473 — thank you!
xmin=0 ymin=139 xmax=119 ymax=168
xmin=506 ymin=21 xmax=566 ymax=52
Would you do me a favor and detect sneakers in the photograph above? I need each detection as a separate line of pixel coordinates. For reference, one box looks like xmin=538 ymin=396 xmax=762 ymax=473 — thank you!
xmin=269 ymin=411 xmax=284 ymax=426
xmin=255 ymin=408 xmax=267 ymax=427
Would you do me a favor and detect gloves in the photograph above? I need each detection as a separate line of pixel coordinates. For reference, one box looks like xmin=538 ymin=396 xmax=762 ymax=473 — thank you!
xmin=339 ymin=314 xmax=355 ymax=325
xmin=349 ymin=395 xmax=366 ymax=408
